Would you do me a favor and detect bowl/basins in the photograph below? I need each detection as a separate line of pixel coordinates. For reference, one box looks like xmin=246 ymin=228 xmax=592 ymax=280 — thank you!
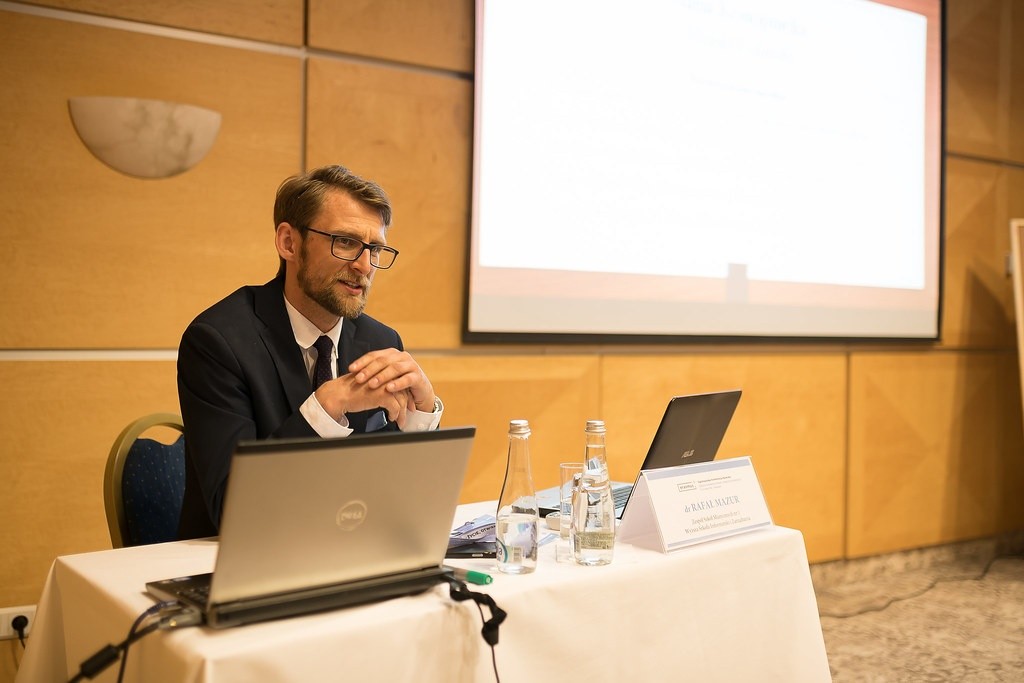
xmin=546 ymin=512 xmax=560 ymax=531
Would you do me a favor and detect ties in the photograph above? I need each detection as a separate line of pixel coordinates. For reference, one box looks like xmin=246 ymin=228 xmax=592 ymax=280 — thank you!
xmin=312 ymin=336 xmax=333 ymax=392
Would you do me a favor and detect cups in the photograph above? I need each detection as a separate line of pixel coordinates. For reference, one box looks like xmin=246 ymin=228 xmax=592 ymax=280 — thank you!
xmin=560 ymin=463 xmax=584 ymax=541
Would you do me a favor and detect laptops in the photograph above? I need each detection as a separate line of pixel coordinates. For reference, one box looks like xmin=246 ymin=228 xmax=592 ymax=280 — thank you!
xmin=512 ymin=390 xmax=742 ymax=519
xmin=148 ymin=426 xmax=475 ymax=630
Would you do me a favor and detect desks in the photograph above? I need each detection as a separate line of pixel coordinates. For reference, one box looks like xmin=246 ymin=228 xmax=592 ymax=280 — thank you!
xmin=14 ymin=499 xmax=834 ymax=683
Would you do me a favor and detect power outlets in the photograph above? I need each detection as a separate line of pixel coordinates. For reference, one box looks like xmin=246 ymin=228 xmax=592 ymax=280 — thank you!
xmin=0 ymin=604 xmax=37 ymax=640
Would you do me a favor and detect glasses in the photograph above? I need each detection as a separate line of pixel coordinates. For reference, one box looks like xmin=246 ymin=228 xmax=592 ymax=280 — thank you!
xmin=297 ymin=227 xmax=399 ymax=269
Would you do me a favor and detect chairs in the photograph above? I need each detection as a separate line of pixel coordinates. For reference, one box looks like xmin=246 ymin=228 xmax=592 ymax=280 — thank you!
xmin=102 ymin=413 xmax=185 ymax=552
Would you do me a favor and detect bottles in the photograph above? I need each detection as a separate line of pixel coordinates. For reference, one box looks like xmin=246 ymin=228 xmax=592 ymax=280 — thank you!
xmin=569 ymin=474 xmax=582 ymax=557
xmin=495 ymin=420 xmax=538 ymax=575
xmin=574 ymin=420 xmax=615 ymax=566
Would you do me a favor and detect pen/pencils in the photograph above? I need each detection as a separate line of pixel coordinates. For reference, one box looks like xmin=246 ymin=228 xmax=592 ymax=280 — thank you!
xmin=441 ymin=565 xmax=493 ymax=586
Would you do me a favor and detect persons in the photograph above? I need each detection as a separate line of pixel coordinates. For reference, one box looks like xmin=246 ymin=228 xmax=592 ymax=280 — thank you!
xmin=176 ymin=166 xmax=445 ymax=539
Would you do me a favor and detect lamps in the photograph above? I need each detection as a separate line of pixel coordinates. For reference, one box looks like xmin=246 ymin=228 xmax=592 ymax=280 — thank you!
xmin=68 ymin=95 xmax=222 ymax=180
